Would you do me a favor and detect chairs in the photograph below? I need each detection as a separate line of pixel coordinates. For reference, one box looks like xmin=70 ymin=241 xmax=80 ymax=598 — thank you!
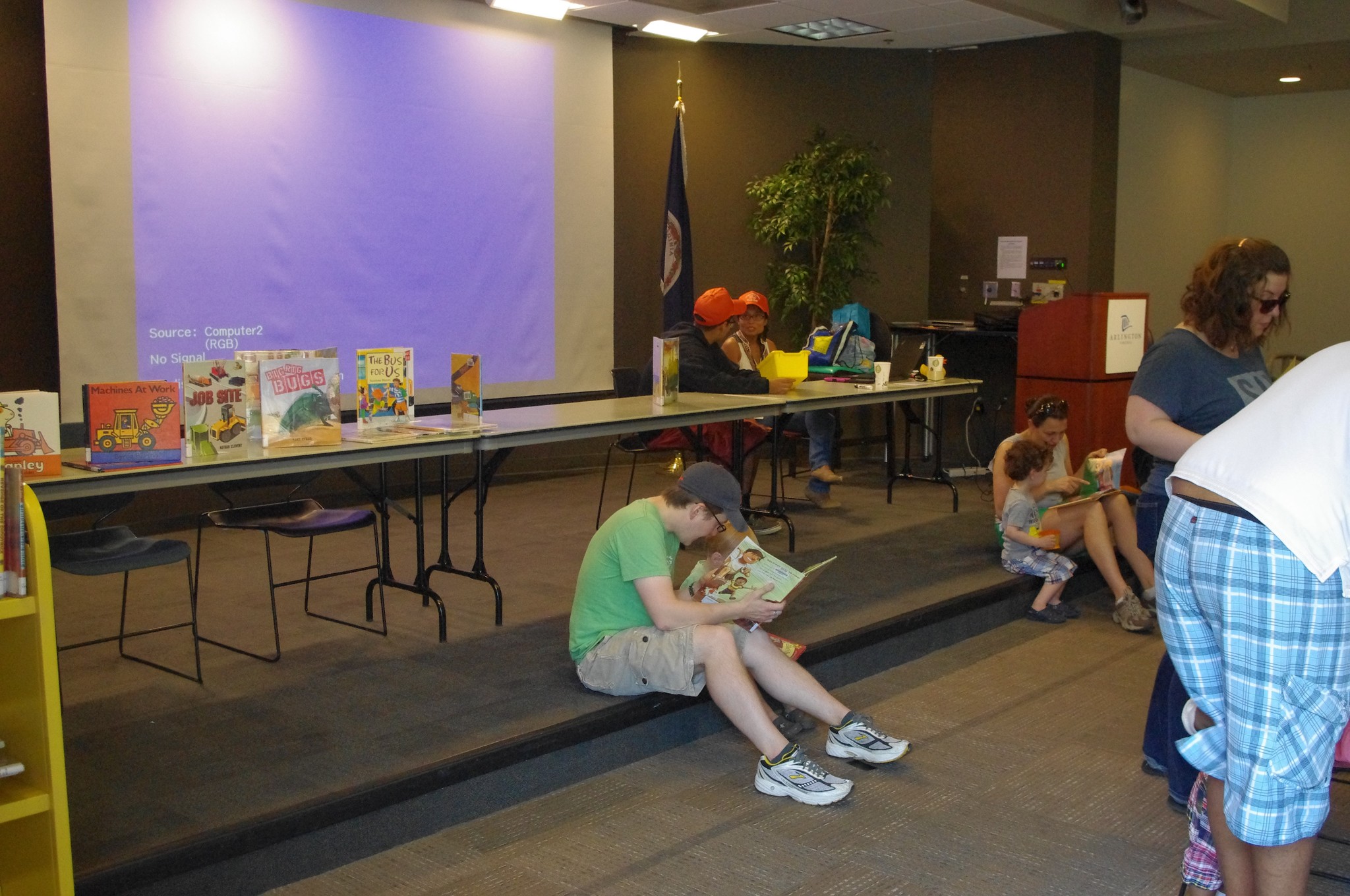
xmin=193 ymin=470 xmax=388 ymax=664
xmin=788 ymin=311 xmax=896 ymax=479
xmin=750 ymin=430 xmax=809 ymax=511
xmin=596 ymin=368 xmax=686 ymax=530
xmin=39 ymin=492 xmax=204 ymax=685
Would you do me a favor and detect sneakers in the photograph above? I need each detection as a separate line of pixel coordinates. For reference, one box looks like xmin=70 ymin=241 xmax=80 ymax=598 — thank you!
xmin=1138 ymin=587 xmax=1157 ymax=617
xmin=755 ymin=744 xmax=854 ymax=806
xmin=745 ymin=512 xmax=782 ymax=536
xmin=826 ymin=709 xmax=912 ymax=763
xmin=1112 ymin=586 xmax=1153 ymax=630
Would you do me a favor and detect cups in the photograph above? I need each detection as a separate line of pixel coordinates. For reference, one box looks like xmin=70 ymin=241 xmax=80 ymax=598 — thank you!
xmin=928 ymin=356 xmax=944 ymax=370
xmin=874 ymin=362 xmax=892 ymax=387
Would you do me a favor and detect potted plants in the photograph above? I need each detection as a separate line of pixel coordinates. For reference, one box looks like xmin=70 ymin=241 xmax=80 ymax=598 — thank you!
xmin=746 ymin=123 xmax=892 ymax=469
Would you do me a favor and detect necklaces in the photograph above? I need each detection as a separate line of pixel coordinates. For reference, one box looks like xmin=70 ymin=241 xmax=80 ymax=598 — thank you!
xmin=1229 ymin=343 xmax=1237 ymax=352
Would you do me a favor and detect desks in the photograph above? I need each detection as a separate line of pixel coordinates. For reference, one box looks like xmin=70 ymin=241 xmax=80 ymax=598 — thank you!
xmin=891 ymin=319 xmax=1018 ymax=458
xmin=721 ymin=376 xmax=984 ymax=554
xmin=1 ymin=392 xmax=784 ymax=685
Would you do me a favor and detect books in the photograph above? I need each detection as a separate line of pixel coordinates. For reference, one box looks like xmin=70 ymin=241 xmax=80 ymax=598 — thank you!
xmin=182 ymin=347 xmax=342 ymax=458
xmin=1048 ymin=448 xmax=1141 ymax=510
xmin=0 ymin=390 xmax=61 ymax=602
xmin=692 ymin=536 xmax=838 ymax=633
xmin=61 ymin=380 xmax=182 ymax=473
xmin=0 ymin=740 xmax=26 ymax=778
xmin=341 ymin=346 xmax=498 ymax=444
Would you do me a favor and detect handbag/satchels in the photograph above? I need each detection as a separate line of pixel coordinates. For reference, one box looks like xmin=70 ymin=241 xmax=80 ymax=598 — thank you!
xmin=801 ymin=302 xmax=876 ymax=373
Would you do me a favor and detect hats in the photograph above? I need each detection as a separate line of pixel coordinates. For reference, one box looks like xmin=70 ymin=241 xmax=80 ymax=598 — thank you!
xmin=693 ymin=287 xmax=747 ymax=326
xmin=739 ymin=291 xmax=769 ymax=315
xmin=677 ymin=462 xmax=750 ymax=532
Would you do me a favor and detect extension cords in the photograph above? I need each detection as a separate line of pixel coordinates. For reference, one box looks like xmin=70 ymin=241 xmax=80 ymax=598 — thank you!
xmin=949 ymin=467 xmax=986 ymax=477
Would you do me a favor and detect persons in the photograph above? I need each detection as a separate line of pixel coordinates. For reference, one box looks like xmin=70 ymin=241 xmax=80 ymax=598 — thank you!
xmin=1179 ymin=697 xmax=1224 ymax=896
xmin=1001 ymin=440 xmax=1081 ymax=624
xmin=719 ymin=290 xmax=843 ymax=508
xmin=659 ymin=99 xmax=695 ymax=332
xmin=679 ymin=520 xmax=760 ymax=590
xmin=567 ymin=461 xmax=914 ymax=806
xmin=1154 ymin=339 xmax=1350 ymax=896
xmin=634 ymin=287 xmax=795 ymax=537
xmin=1124 ymin=237 xmax=1293 ymax=814
xmin=987 ymin=394 xmax=1156 ymax=630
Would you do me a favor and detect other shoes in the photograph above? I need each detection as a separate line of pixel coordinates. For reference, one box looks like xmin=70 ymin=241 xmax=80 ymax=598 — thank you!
xmin=1141 ymin=760 xmax=1168 ymax=776
xmin=804 ymin=486 xmax=842 ymax=509
xmin=810 ymin=468 xmax=840 ymax=482
xmin=1167 ymin=796 xmax=1188 ymax=815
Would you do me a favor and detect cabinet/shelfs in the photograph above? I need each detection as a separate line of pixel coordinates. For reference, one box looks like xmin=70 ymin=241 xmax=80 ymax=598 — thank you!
xmin=0 ymin=482 xmax=74 ymax=896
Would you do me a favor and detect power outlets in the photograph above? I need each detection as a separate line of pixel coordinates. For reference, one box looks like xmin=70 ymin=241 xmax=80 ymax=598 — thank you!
xmin=1030 ymin=258 xmax=1067 ymax=270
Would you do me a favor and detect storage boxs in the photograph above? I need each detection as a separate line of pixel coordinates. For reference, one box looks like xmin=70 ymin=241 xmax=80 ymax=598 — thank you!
xmin=756 ymin=350 xmax=811 ymax=389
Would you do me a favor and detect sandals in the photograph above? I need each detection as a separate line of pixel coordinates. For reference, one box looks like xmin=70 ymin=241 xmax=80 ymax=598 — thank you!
xmin=772 ymin=715 xmax=802 ymax=739
xmin=1025 ymin=606 xmax=1066 ymax=624
xmin=1047 ymin=600 xmax=1077 ymax=618
xmin=781 ymin=708 xmax=814 ymax=730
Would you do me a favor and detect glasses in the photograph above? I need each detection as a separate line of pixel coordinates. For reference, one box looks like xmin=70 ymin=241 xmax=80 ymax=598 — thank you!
xmin=739 ymin=313 xmax=766 ymax=321
xmin=1247 ymin=290 xmax=1291 ymax=314
xmin=720 ymin=319 xmax=736 ymax=327
xmin=1031 ymin=400 xmax=1069 ymax=418
xmin=695 ymin=501 xmax=726 ymax=532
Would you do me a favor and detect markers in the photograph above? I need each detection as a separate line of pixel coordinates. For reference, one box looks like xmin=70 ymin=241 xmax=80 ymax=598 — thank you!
xmin=854 ymin=384 xmax=872 ymax=389
